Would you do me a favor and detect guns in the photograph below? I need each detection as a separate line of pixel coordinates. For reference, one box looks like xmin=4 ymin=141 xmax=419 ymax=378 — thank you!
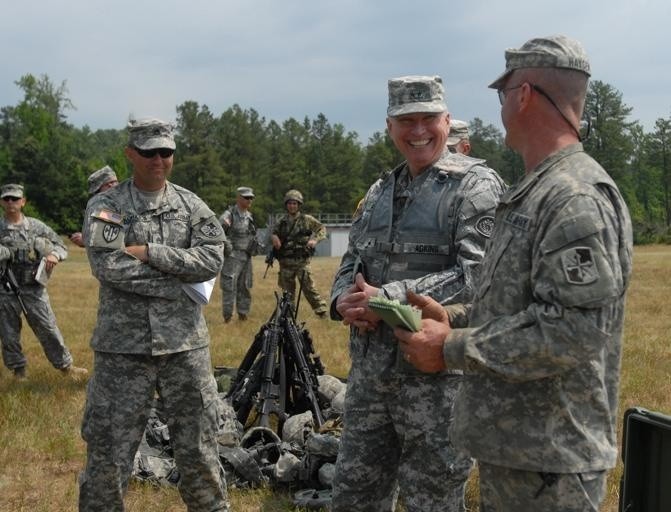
xmin=222 ymin=288 xmax=325 ymax=431
xmin=4 ymin=243 xmax=29 ymax=318
xmin=261 ymin=218 xmax=302 ymax=280
xmin=244 ymin=237 xmax=260 ymax=257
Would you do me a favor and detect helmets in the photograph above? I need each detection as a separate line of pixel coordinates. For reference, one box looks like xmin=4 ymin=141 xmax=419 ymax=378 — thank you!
xmin=284 ymin=189 xmax=303 ymax=204
xmin=318 ymin=373 xmax=343 ymax=406
xmin=283 ymin=410 xmax=314 ymax=448
xmin=330 ymin=390 xmax=346 ymax=414
xmin=240 ymin=426 xmax=280 ymax=474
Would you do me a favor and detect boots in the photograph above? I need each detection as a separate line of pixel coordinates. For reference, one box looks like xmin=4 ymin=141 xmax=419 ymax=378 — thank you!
xmin=62 ymin=364 xmax=89 ymax=377
xmin=12 ymin=366 xmax=27 ymax=382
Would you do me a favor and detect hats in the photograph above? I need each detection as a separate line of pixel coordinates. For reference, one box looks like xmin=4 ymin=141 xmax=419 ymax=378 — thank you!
xmin=387 ymin=76 xmax=447 ymax=117
xmin=87 ymin=165 xmax=117 ymax=194
xmin=1 ymin=184 xmax=24 ymax=198
xmin=237 ymin=187 xmax=255 ymax=197
xmin=488 ymin=33 xmax=591 ymax=90
xmin=445 ymin=119 xmax=468 ymax=146
xmin=128 ymin=119 xmax=177 ymax=150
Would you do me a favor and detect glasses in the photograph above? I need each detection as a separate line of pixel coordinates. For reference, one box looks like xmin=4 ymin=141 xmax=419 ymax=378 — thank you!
xmin=3 ymin=196 xmax=21 ymax=201
xmin=497 ymin=84 xmax=533 ymax=104
xmin=449 ymin=148 xmax=457 ymax=153
xmin=134 ymin=147 xmax=174 ymax=158
xmin=240 ymin=195 xmax=253 ymax=200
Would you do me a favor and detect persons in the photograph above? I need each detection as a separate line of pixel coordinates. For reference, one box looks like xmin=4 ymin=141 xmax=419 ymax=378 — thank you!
xmin=77 ymin=119 xmax=234 ymax=512
xmin=393 ymin=32 xmax=634 ymax=510
xmin=324 ymin=74 xmax=508 ymax=511
xmin=264 ymin=188 xmax=331 ymax=320
xmin=447 ymin=119 xmax=472 ymax=154
xmin=0 ymin=183 xmax=89 ymax=384
xmin=218 ymin=185 xmax=257 ymax=325
xmin=69 ymin=165 xmax=120 ymax=248
